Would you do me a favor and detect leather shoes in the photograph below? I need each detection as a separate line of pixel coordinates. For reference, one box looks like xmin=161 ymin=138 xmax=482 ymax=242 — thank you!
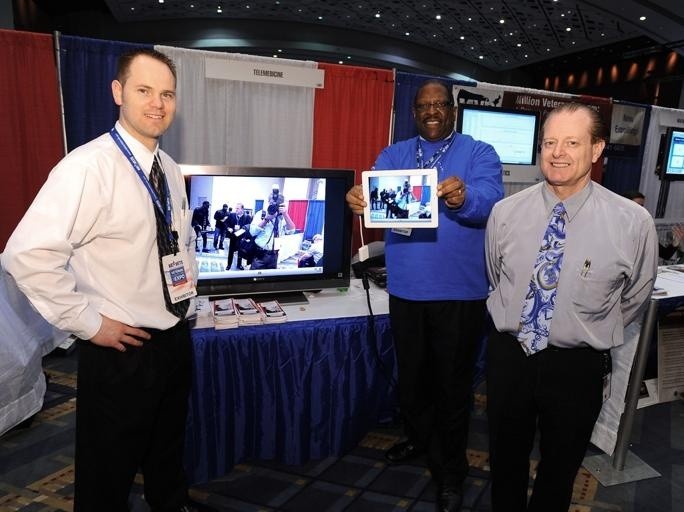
xmin=384 ymin=439 xmax=429 ymax=464
xmin=436 ymin=484 xmax=462 ymax=512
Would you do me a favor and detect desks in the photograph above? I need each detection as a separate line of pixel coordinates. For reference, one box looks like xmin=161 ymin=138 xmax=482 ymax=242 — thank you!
xmin=181 ymin=276 xmax=398 ymax=482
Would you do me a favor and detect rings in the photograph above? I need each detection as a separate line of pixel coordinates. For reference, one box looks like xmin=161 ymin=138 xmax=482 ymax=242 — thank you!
xmin=459 ymin=189 xmax=462 ymax=195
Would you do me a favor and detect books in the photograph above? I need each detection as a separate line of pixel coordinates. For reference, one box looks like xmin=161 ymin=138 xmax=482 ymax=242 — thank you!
xmin=212 ymin=298 xmax=290 ymax=332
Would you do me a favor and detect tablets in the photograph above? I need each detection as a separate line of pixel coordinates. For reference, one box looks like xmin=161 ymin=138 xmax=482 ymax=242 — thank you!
xmin=360 ymin=170 xmax=439 ymax=228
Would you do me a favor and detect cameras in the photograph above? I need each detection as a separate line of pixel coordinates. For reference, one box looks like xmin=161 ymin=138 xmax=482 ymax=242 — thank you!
xmin=268 ymin=189 xmax=282 ymax=216
xmin=402 ymin=181 xmax=411 ymax=194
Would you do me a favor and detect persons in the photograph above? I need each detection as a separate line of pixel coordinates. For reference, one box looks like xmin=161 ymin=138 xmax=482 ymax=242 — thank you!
xmin=483 ymin=102 xmax=659 ymax=512
xmin=0 ymin=48 xmax=198 ymax=510
xmin=345 ymin=78 xmax=506 ymax=510
xmin=193 ymin=194 xmax=325 ymax=271
xmin=620 ymin=191 xmax=684 ymax=262
xmin=370 ymin=183 xmax=432 ymax=220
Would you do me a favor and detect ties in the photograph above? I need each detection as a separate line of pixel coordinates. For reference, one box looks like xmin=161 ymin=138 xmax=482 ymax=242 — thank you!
xmin=518 ymin=203 xmax=566 ymax=358
xmin=149 ymin=155 xmax=191 ymax=320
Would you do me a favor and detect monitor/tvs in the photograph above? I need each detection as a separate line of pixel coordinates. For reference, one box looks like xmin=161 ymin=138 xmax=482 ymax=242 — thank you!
xmin=656 ymin=127 xmax=684 ymax=181
xmin=458 ymin=106 xmax=540 ymax=167
xmin=175 ymin=164 xmax=356 ymax=304
